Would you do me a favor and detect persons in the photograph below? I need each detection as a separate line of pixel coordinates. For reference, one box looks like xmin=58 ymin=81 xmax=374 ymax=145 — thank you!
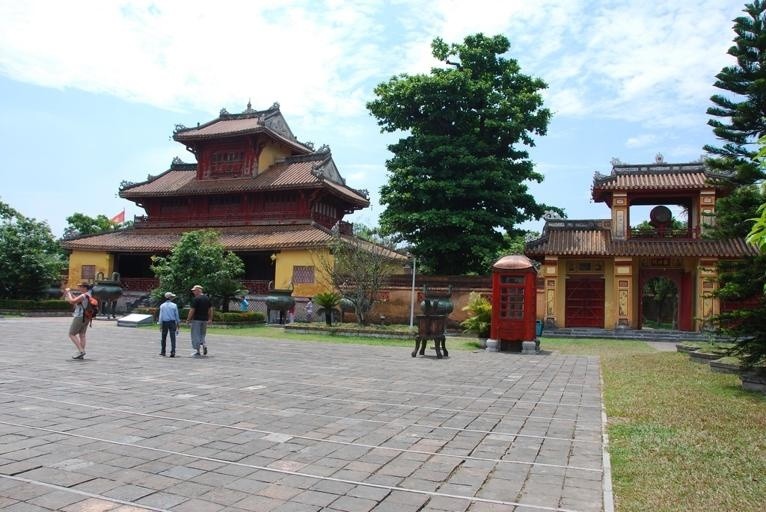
xmin=63 ymin=280 xmax=92 ymax=359
xmin=303 ymin=296 xmax=313 ymax=323
xmin=288 ymin=303 xmax=297 ymax=324
xmin=185 ymin=284 xmax=213 ymax=358
xmin=239 ymin=295 xmax=249 ymax=313
xmin=155 ymin=291 xmax=181 ymax=358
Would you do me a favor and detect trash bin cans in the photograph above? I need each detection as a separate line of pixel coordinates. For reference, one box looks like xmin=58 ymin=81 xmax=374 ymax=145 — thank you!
xmin=536 ymin=319 xmax=543 ymax=337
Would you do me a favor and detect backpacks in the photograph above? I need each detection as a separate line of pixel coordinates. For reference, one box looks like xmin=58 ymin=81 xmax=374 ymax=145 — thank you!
xmin=77 ymin=293 xmax=99 ymax=318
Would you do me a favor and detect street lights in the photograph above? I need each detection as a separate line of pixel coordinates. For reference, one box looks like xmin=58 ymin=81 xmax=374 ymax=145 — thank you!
xmin=406 ymin=250 xmax=422 ymax=327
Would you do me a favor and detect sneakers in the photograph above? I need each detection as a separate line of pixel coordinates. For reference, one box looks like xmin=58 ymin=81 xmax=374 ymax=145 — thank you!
xmin=160 ymin=351 xmax=175 ymax=357
xmin=194 ymin=345 xmax=207 ymax=357
xmin=72 ymin=351 xmax=86 ymax=359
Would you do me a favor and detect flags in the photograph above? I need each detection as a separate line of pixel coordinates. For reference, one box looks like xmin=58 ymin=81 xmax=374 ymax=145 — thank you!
xmin=109 ymin=210 xmax=125 ymax=224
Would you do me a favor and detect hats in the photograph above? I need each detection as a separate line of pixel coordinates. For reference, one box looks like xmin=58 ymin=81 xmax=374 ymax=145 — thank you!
xmin=191 ymin=285 xmax=203 ymax=290
xmin=77 ymin=279 xmax=89 ymax=285
xmin=165 ymin=292 xmax=175 ymax=299
